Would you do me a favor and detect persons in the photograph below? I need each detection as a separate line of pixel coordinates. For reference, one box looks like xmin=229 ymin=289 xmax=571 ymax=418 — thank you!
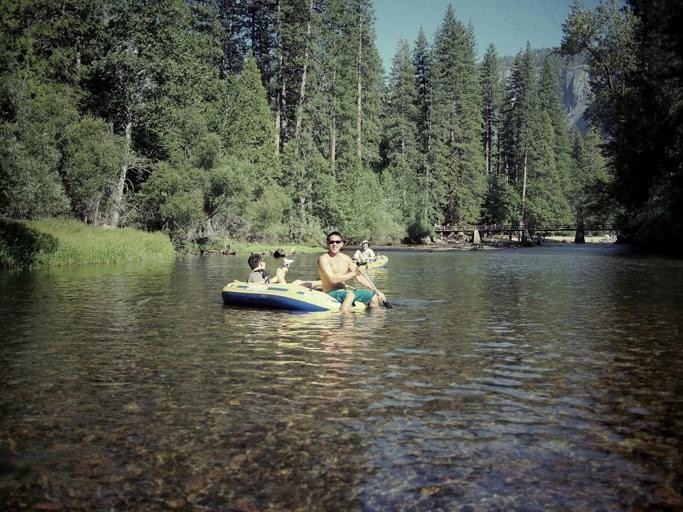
xmin=247 ymin=254 xmax=323 ymax=290
xmin=352 ymin=239 xmax=376 ymax=266
xmin=317 ymin=231 xmax=387 ymax=314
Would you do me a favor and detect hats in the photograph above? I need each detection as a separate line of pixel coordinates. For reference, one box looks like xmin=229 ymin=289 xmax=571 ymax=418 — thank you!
xmin=362 ymin=241 xmax=370 ymax=246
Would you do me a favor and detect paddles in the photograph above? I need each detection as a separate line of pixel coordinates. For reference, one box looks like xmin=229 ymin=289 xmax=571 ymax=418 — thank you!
xmin=356 ymin=262 xmax=392 ymax=309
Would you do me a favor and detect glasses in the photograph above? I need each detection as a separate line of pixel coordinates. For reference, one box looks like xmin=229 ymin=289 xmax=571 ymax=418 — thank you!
xmin=330 ymin=241 xmax=341 ymax=244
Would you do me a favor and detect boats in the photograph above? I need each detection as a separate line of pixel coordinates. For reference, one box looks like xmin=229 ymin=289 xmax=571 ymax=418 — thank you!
xmin=353 ymin=253 xmax=389 ymax=273
xmin=221 ymin=277 xmax=375 ymax=314
